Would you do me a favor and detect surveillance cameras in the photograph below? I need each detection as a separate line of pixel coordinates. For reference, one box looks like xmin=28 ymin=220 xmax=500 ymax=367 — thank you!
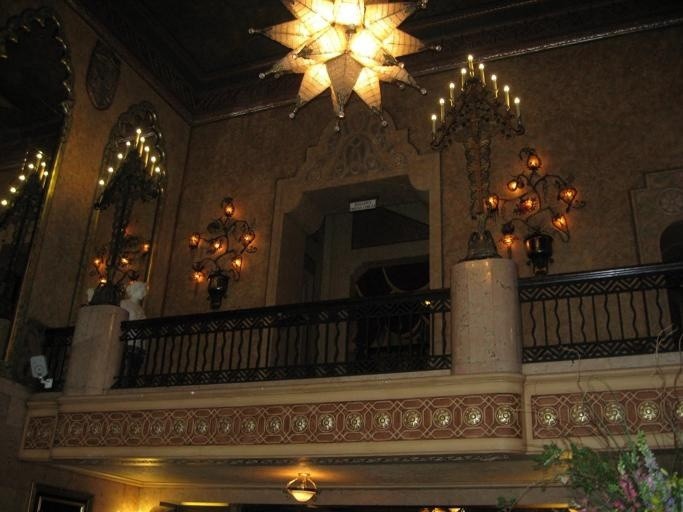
xmin=29 ymin=355 xmax=49 ymax=381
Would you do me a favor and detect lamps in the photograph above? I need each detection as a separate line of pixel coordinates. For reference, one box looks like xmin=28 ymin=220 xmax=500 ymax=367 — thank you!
xmin=244 ymin=2 xmax=443 ymax=134
xmin=481 ymin=146 xmax=579 ymax=272
xmin=188 ymin=196 xmax=259 ymax=311
